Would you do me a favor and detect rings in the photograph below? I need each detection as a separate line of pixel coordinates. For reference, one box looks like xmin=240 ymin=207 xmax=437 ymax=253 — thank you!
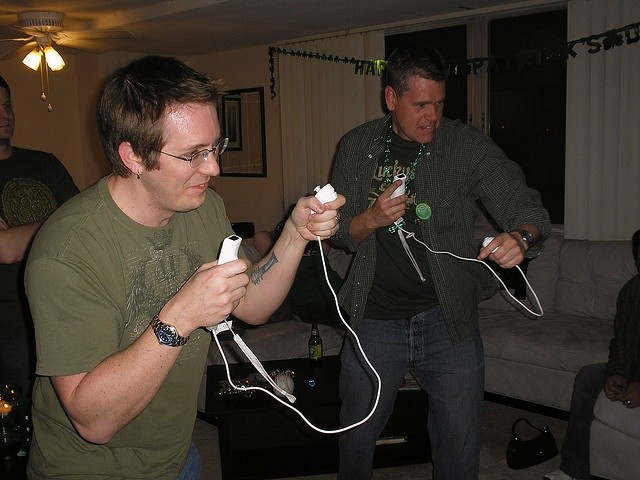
xmin=626 ymin=400 xmax=631 ymax=405
xmin=608 ymin=381 xmax=615 ymax=384
xmin=335 ymin=216 xmax=341 ymax=224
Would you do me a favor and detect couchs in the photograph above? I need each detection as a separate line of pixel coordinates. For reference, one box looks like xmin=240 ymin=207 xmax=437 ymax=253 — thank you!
xmin=194 ymin=233 xmax=640 ymax=479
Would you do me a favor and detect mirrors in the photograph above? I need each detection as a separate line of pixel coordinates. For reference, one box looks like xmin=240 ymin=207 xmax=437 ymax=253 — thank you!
xmin=215 ymin=85 xmax=269 ymax=179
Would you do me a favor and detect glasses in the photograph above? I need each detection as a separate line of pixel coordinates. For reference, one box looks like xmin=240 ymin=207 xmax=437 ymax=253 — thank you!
xmin=145 ymin=136 xmax=228 ymax=169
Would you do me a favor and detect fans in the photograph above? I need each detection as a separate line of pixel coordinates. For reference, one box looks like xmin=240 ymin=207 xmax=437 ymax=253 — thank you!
xmin=0 ymin=10 xmax=133 ymax=59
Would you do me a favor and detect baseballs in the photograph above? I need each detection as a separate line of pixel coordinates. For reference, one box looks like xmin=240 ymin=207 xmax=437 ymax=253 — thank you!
xmin=272 ymin=375 xmax=295 ymax=398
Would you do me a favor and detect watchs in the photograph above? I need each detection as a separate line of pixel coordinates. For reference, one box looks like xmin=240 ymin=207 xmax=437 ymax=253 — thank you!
xmin=509 ymin=228 xmax=535 ymax=251
xmin=149 ymin=315 xmax=190 ymax=348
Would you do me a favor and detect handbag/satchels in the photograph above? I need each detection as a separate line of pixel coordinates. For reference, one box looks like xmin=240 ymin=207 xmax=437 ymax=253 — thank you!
xmin=506 ymin=418 xmax=559 ymax=469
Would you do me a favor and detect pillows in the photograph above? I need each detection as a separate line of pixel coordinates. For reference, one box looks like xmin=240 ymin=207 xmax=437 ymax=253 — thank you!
xmin=478 ymin=233 xmax=564 ymax=315
xmin=556 ymin=239 xmax=638 ymax=323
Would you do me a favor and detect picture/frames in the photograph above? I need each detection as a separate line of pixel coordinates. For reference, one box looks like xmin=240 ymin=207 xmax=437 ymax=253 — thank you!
xmin=220 ymin=98 xmax=245 ymax=153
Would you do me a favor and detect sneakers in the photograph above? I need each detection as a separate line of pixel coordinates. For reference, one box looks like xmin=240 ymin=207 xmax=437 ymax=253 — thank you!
xmin=543 ymin=468 xmax=574 ymax=479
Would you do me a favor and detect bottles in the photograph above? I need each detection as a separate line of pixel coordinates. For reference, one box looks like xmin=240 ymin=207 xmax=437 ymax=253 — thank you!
xmin=308 ymin=324 xmax=323 ymax=362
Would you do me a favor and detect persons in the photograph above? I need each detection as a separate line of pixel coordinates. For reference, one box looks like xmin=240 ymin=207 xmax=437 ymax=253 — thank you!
xmin=323 ymin=43 xmax=552 ymax=479
xmin=253 ymin=193 xmax=343 ymax=320
xmin=0 ymin=75 xmax=82 ymax=404
xmin=541 ymin=228 xmax=639 ymax=479
xmin=23 ymin=54 xmax=346 ymax=479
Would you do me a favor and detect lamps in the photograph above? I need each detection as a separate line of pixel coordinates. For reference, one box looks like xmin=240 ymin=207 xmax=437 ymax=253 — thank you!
xmin=22 ymin=36 xmax=67 ymax=111
